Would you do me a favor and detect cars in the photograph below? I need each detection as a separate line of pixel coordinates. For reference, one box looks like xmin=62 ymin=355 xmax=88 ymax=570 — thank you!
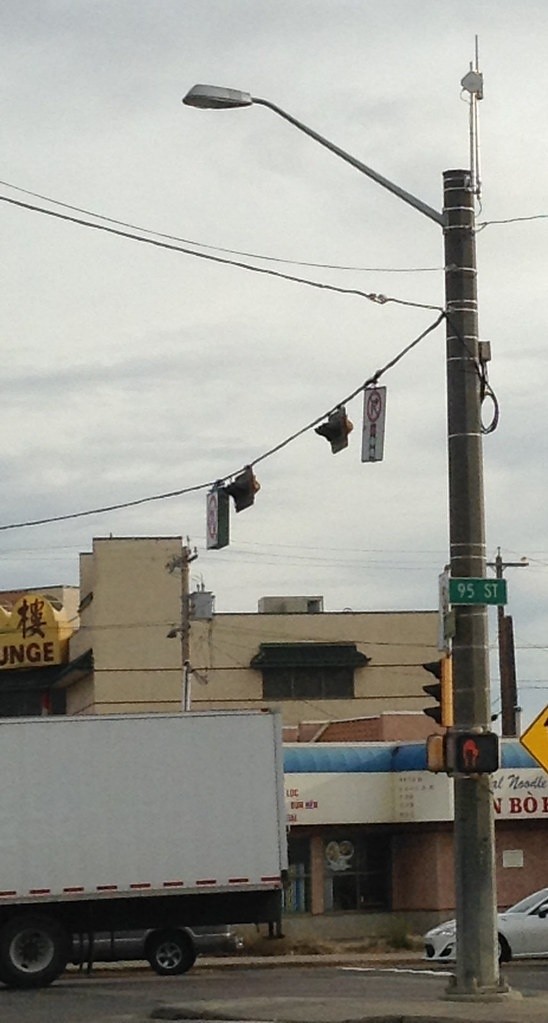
xmin=68 ymin=920 xmax=271 ymax=977
xmin=421 ymin=887 xmax=548 ymax=968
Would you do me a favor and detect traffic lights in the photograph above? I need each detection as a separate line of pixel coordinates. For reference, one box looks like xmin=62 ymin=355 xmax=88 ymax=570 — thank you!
xmin=221 ymin=469 xmax=256 ymax=516
xmin=315 ymin=407 xmax=352 ymax=457
xmin=456 ymin=733 xmax=499 ymax=772
xmin=421 ymin=657 xmax=452 ymax=727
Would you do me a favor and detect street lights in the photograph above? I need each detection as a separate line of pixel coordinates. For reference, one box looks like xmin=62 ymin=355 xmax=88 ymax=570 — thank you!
xmin=180 ymin=82 xmax=513 ymax=999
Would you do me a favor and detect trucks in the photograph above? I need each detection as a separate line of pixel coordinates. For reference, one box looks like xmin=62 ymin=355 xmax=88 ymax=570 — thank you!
xmin=0 ymin=706 xmax=288 ymax=993
